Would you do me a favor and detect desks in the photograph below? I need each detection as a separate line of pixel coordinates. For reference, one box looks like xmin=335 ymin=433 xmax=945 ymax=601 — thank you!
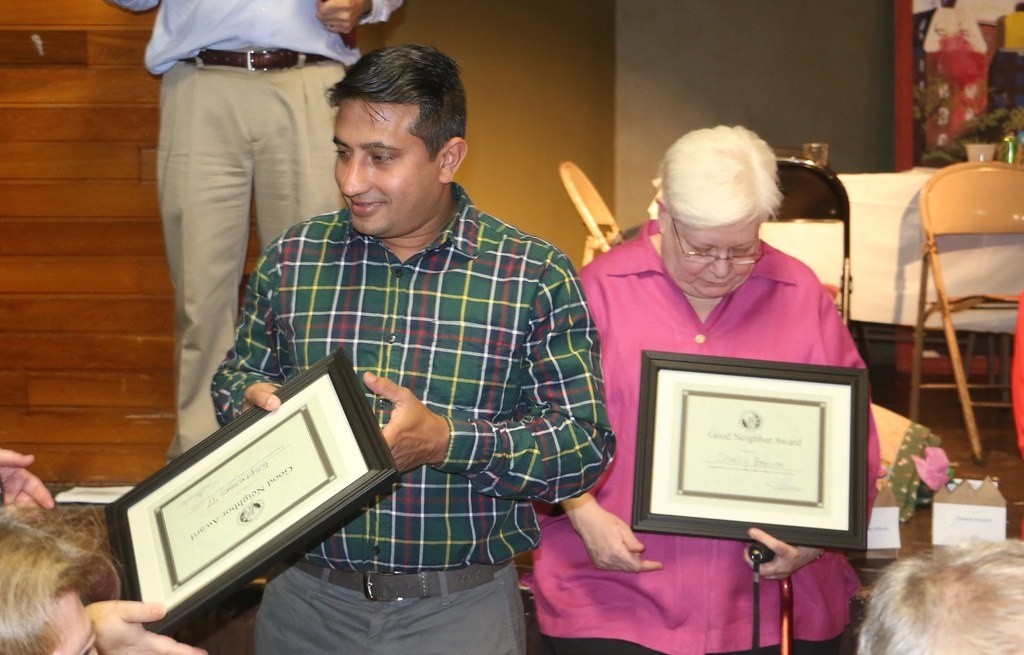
xmin=646 ymin=171 xmax=1024 ymax=410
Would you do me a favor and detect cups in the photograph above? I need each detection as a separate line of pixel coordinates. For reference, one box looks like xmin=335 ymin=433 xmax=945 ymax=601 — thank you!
xmin=962 ymin=144 xmax=995 ymax=164
xmin=802 ymin=143 xmax=829 ymax=168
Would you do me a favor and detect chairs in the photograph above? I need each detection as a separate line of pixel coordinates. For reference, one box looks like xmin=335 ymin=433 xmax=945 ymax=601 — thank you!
xmin=908 ymin=162 xmax=1022 ymax=464
xmin=558 ymin=160 xmax=624 ymax=268
xmin=761 ymin=156 xmax=882 ymax=399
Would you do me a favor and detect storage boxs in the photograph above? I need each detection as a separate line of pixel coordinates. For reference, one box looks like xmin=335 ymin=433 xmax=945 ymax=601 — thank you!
xmin=867 ymin=505 xmax=902 ymax=549
xmin=931 ymin=475 xmax=1007 ymax=548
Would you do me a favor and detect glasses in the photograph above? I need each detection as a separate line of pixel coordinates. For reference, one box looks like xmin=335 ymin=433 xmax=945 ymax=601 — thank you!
xmin=672 ymin=216 xmax=762 ymax=264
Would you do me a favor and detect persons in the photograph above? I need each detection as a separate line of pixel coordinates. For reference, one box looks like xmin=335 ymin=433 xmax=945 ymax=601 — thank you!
xmin=210 ymin=43 xmax=616 ymax=655
xmin=856 ymin=538 xmax=1024 ymax=655
xmin=519 ymin=125 xmax=881 ymax=655
xmin=108 ymin=0 xmax=405 ymax=464
xmin=0 ymin=445 xmax=209 ymax=655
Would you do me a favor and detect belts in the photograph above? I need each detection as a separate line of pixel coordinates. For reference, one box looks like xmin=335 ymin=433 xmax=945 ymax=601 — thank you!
xmin=281 ymin=550 xmax=514 ymax=603
xmin=178 ymin=50 xmax=331 ymax=72
